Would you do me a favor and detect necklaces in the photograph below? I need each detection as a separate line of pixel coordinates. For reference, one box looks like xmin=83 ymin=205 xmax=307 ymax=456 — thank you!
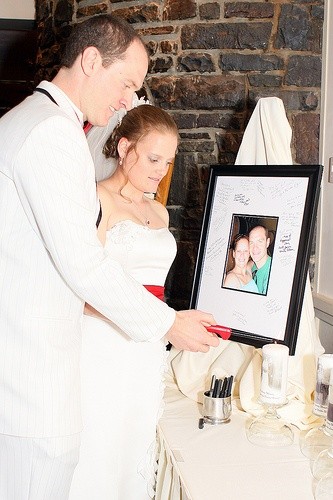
xmin=123 ymin=192 xmax=151 ymax=226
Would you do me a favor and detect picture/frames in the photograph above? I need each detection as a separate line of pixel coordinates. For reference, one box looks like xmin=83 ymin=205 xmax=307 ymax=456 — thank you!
xmin=189 ymin=164 xmax=324 ymax=355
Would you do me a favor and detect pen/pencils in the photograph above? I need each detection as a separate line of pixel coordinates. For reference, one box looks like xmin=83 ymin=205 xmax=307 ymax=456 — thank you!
xmin=209 ymin=375 xmax=234 ymax=398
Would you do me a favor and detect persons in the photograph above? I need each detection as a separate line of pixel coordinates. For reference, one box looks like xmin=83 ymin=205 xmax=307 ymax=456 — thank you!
xmin=249 ymin=225 xmax=272 ymax=295
xmin=1 ymin=13 xmax=220 ymax=500
xmin=223 ymin=233 xmax=259 ymax=293
xmin=71 ymin=104 xmax=178 ymax=500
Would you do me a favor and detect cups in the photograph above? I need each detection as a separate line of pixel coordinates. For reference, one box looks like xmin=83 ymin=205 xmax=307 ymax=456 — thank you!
xmin=202 ymin=391 xmax=232 ymax=425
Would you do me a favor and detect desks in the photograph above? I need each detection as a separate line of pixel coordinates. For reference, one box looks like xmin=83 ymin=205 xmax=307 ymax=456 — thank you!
xmin=154 ymin=350 xmax=333 ymax=499
